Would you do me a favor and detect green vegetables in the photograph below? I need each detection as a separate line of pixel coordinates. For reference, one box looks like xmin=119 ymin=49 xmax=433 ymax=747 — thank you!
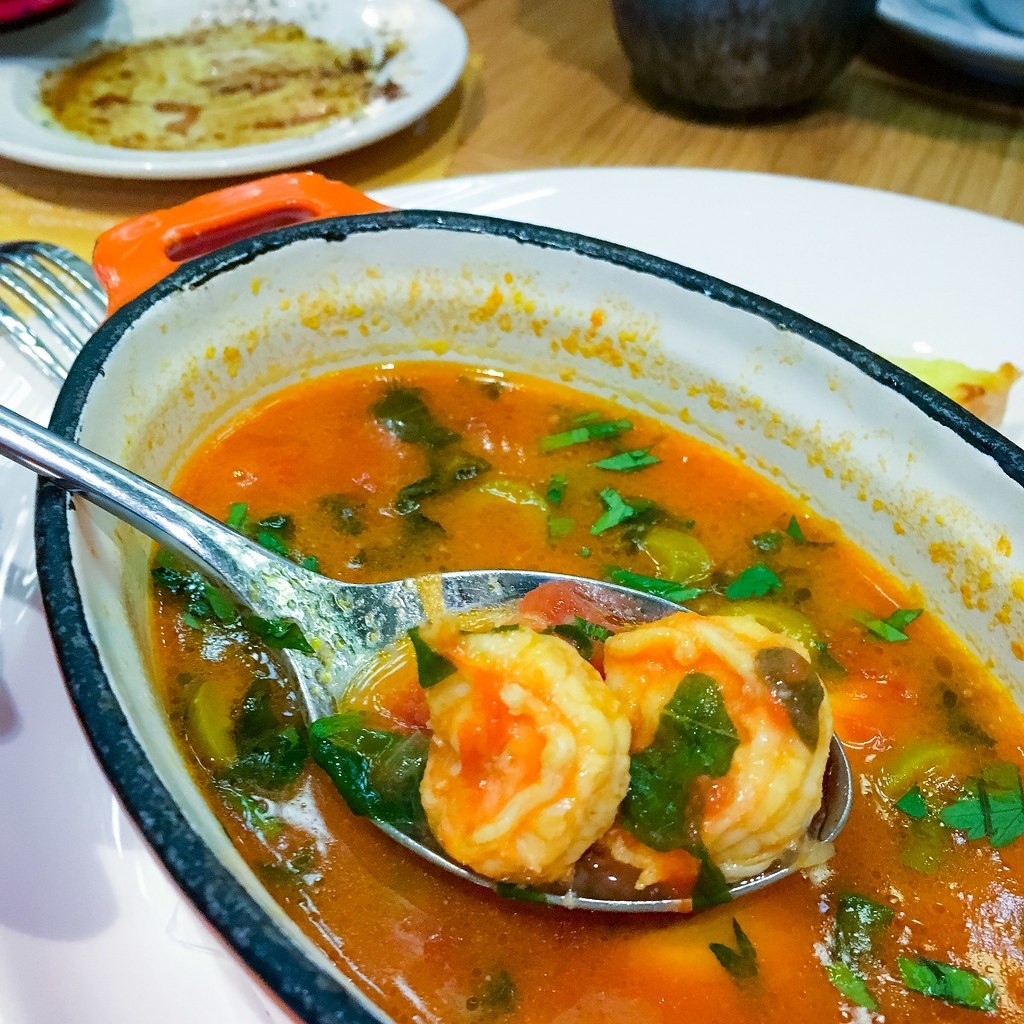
xmin=148 ymin=381 xmax=1024 ymax=1024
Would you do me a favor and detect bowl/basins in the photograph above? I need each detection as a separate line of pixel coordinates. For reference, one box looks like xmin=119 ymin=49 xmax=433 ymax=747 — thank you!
xmin=35 ymin=210 xmax=1024 ymax=1024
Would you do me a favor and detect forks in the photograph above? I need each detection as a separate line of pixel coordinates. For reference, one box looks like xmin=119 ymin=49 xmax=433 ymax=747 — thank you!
xmin=0 ymin=237 xmax=111 ymax=388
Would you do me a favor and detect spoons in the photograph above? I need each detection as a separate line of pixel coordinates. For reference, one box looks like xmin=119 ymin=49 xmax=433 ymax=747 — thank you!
xmin=0 ymin=404 xmax=854 ymax=914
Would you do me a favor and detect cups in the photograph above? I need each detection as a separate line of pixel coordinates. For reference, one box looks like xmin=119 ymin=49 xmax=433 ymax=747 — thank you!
xmin=610 ymin=0 xmax=878 ymax=126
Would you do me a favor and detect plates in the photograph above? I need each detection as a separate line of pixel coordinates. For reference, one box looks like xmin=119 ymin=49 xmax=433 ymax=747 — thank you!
xmin=0 ymin=0 xmax=470 ymax=182
xmin=0 ymin=167 xmax=1023 ymax=1024
